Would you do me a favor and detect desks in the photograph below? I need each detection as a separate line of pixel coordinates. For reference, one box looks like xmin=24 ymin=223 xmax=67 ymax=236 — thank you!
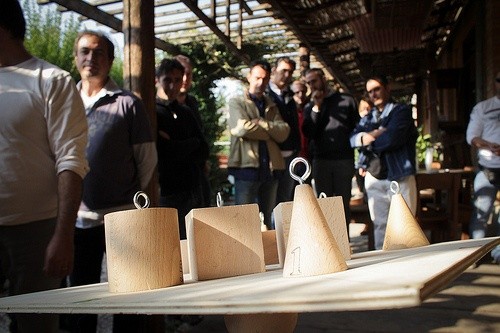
xmin=0 ymin=236 xmax=500 ymax=332
xmin=367 ymin=167 xmax=474 ymax=250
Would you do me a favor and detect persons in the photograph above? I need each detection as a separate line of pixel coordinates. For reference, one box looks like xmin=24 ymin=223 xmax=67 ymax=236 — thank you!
xmin=466 ymin=71 xmax=500 ymax=269
xmin=301 ymin=68 xmax=362 ymax=243
xmin=60 ymin=30 xmax=157 ymax=333
xmin=350 ymin=73 xmax=418 ymax=251
xmin=264 ymin=56 xmax=300 ymax=230
xmin=291 ymin=79 xmax=308 ymax=184
xmin=155 ymin=59 xmax=210 ymax=240
xmin=0 ymin=0 xmax=90 ymax=333
xmin=170 ymin=54 xmax=209 ymax=178
xmin=348 ymin=95 xmax=374 ymax=235
xmin=227 ymin=59 xmax=291 ymax=230
